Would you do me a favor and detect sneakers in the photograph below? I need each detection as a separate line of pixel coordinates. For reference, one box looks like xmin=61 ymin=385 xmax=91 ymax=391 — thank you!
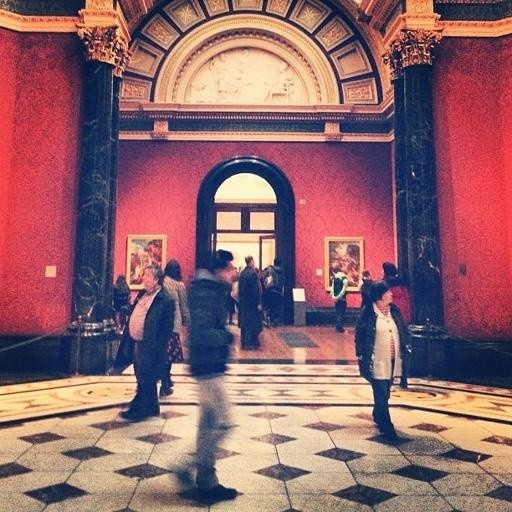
xmin=160 ymin=380 xmax=174 ymax=396
xmin=197 ymin=484 xmax=237 ymax=503
xmin=399 ymin=379 xmax=408 ymax=388
xmin=120 ymin=409 xmax=160 ymax=420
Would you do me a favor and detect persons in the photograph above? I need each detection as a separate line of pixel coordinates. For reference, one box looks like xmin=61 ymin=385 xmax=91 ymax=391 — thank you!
xmin=229 ymin=253 xmax=286 ymax=350
xmin=331 ymin=267 xmax=349 ymax=334
xmin=354 ymin=279 xmax=410 ymax=443
xmin=171 ymin=248 xmax=241 ymax=498
xmin=112 ymin=264 xmax=176 ymax=420
xmin=358 ymin=269 xmax=374 ymax=306
xmin=158 ymin=258 xmax=191 ymax=397
xmin=114 ymin=273 xmax=132 ymax=331
xmin=381 ymin=260 xmax=414 ymax=390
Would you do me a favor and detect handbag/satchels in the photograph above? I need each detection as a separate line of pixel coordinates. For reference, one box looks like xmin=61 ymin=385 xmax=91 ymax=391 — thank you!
xmin=231 ymin=281 xmax=240 ymax=303
xmin=266 ymin=276 xmax=273 ymax=288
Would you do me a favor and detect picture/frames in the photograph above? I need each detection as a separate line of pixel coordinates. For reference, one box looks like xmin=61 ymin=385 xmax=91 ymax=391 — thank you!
xmin=125 ymin=235 xmax=167 ymax=290
xmin=324 ymin=236 xmax=365 ymax=292
